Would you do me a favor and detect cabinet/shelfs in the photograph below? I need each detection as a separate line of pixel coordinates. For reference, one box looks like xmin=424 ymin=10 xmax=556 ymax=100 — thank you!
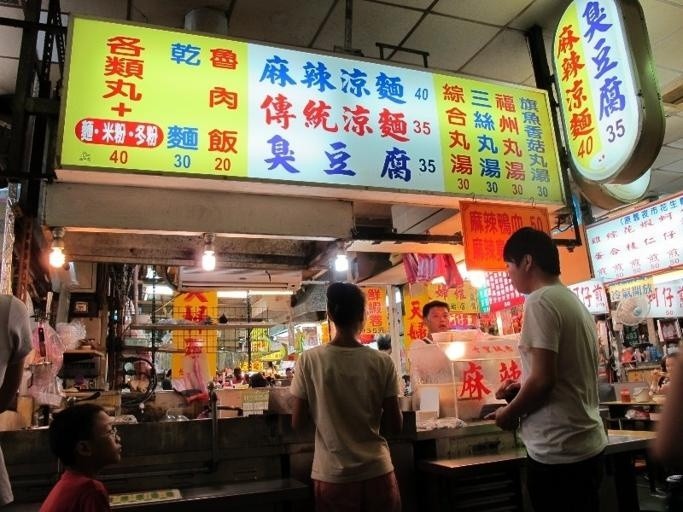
xmin=58 ymin=341 xmax=105 ymax=397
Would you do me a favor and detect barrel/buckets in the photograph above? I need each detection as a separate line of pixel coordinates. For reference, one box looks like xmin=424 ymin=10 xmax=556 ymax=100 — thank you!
xmin=613 ymin=380 xmax=651 ymax=401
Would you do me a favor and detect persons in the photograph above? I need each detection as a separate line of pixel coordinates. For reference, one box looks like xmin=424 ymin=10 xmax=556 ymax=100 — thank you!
xmin=410 ymin=301 xmax=449 ymax=349
xmin=162 ymin=370 xmax=172 ymax=390
xmin=495 ymin=227 xmax=610 ymax=512
xmin=37 ymin=404 xmax=122 ymax=512
xmin=285 ymin=282 xmax=403 ymax=510
xmin=211 ymin=361 xmax=277 ymax=389
xmin=0 ymin=293 xmax=33 ymax=507
xmin=650 ymin=342 xmax=682 ymax=464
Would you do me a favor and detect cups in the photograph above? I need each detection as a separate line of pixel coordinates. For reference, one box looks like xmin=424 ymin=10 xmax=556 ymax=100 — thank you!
xmin=136 ymin=315 xmax=150 ymax=325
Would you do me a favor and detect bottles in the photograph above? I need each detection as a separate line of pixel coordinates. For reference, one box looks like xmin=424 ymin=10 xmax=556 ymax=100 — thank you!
xmin=646 ymin=343 xmax=657 ymax=362
xmin=633 ymin=347 xmax=642 ymax=363
xmin=621 ymin=387 xmax=631 ymax=402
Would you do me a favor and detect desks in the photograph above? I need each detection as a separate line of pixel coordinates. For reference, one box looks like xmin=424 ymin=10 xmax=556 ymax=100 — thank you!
xmin=597 ymin=395 xmax=665 ymax=489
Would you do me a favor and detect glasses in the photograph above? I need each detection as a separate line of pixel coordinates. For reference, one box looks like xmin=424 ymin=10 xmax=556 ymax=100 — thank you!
xmin=93 ymin=424 xmax=118 ymax=445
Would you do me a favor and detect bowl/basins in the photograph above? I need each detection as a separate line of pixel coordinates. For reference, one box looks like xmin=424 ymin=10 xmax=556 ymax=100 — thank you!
xmin=431 ymin=330 xmax=478 ymax=342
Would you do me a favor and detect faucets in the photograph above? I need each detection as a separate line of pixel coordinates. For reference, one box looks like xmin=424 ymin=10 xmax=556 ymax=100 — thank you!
xmin=197 ymin=404 xmax=245 ymax=418
